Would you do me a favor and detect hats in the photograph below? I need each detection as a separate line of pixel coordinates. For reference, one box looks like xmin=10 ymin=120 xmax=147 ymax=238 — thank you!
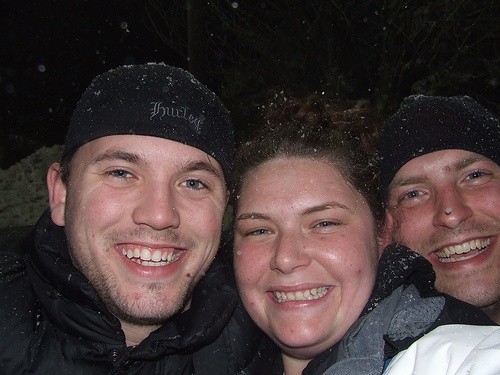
xmin=375 ymin=94 xmax=500 ymax=205
xmin=59 ymin=63 xmax=237 ymax=205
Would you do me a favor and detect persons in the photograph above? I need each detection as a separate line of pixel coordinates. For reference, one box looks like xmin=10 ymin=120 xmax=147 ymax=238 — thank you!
xmin=1 ymin=62 xmax=276 ymax=374
xmin=366 ymin=94 xmax=499 ymax=326
xmin=225 ymin=94 xmax=499 ymax=375
